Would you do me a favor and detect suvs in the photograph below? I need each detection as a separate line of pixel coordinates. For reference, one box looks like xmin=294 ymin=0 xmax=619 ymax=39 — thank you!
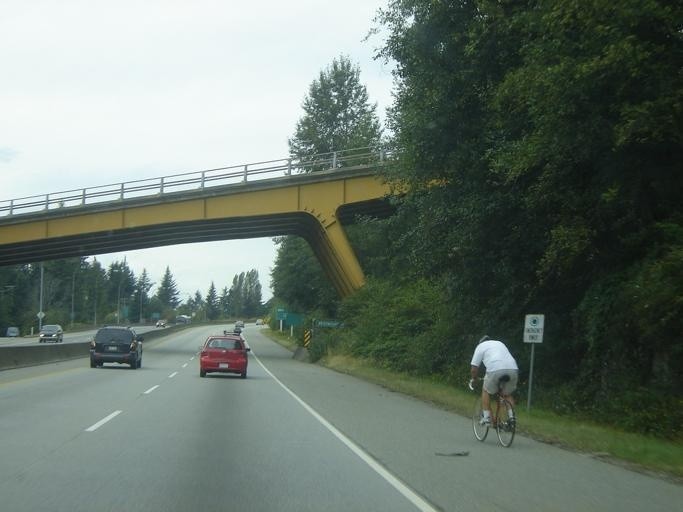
xmin=88 ymin=325 xmax=144 ymax=369
xmin=199 ymin=330 xmax=251 ymax=378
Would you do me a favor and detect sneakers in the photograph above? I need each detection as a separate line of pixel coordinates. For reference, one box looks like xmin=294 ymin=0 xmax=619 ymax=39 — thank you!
xmin=478 ymin=417 xmax=491 ymax=424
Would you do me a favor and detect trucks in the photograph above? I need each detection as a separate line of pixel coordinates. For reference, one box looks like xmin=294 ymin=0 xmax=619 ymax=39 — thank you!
xmin=174 ymin=315 xmax=191 ymax=326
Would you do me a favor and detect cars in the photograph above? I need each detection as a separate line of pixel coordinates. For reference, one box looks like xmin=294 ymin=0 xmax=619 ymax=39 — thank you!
xmin=155 ymin=319 xmax=166 ymax=328
xmin=255 ymin=318 xmax=265 ymax=326
xmin=39 ymin=324 xmax=64 ymax=344
xmin=234 ymin=320 xmax=244 ymax=328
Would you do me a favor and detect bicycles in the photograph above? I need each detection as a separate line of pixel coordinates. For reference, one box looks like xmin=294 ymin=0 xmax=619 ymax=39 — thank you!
xmin=468 ymin=374 xmax=518 ymax=448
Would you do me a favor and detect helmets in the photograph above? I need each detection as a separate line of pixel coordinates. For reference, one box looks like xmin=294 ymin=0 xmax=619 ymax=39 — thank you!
xmin=478 ymin=335 xmax=489 ymax=343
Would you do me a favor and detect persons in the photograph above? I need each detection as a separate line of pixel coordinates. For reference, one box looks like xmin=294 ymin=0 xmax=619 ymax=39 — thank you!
xmin=466 ymin=333 xmax=520 ymax=427
